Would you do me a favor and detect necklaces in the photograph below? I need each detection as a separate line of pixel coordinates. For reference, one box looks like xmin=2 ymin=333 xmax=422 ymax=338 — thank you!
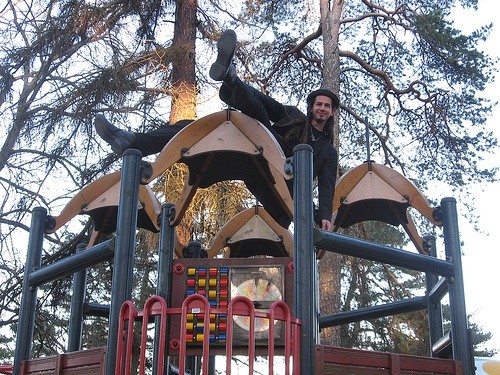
xmin=309 ymin=124 xmax=318 ymax=141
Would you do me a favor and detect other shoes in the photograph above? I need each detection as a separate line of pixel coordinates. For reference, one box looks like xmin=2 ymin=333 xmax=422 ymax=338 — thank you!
xmin=209 ymin=30 xmax=237 ymax=83
xmin=95 ymin=114 xmax=136 ymax=156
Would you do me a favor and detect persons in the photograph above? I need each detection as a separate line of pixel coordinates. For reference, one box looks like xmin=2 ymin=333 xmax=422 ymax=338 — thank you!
xmin=95 ymin=29 xmax=340 ymax=231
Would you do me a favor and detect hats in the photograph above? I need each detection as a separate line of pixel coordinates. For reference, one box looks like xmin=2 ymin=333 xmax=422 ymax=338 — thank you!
xmin=307 ymin=89 xmax=341 ymax=111
